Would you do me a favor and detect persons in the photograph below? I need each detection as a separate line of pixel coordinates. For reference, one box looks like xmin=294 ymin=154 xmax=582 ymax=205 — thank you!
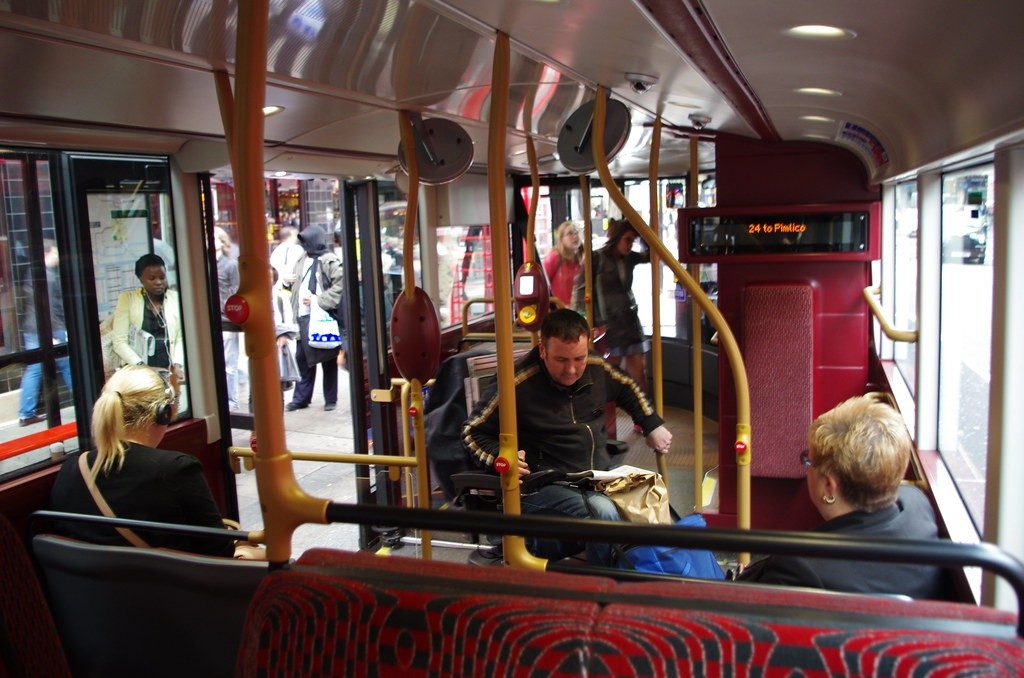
xmin=50 ymin=364 xmax=236 ymax=559
xmin=18 ymin=208 xmax=344 ymax=427
xmin=733 ymin=398 xmax=942 ymax=600
xmin=460 ymin=308 xmax=673 ymax=565
xmin=541 ymin=221 xmax=584 ymax=309
xmin=570 ymin=219 xmax=650 ymax=441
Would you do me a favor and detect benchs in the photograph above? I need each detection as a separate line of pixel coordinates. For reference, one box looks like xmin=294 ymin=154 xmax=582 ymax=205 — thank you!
xmin=0 ymin=504 xmax=1023 ymax=678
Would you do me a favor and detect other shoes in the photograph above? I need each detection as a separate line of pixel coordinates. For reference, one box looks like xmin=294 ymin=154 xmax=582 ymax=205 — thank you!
xmin=323 ymin=402 xmax=336 ymax=411
xmin=19 ymin=415 xmax=46 ymax=426
xmin=286 ymin=402 xmax=308 ymax=411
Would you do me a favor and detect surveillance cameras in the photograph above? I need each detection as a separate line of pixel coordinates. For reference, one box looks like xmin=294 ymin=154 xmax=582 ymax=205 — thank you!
xmin=689 ymin=114 xmax=711 ymax=129
xmin=623 ymin=73 xmax=658 ymax=94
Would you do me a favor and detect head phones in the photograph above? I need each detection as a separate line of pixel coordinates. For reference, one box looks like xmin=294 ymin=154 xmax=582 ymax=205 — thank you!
xmin=156 ymin=376 xmax=176 ymax=425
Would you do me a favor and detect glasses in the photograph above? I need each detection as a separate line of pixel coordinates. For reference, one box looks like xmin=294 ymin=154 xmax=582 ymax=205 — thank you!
xmin=798 ymin=450 xmax=814 ymax=468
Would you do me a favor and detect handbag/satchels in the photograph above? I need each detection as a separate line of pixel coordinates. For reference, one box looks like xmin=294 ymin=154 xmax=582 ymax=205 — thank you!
xmin=308 ymin=295 xmax=342 ymax=349
xmin=620 ymin=515 xmax=726 ymax=582
xmin=100 ymin=313 xmax=142 ymax=374
xmin=570 ymin=249 xmax=608 ymax=327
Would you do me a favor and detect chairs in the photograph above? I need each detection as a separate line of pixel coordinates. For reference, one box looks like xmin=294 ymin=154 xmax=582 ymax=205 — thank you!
xmin=451 ymin=350 xmax=626 ymax=534
